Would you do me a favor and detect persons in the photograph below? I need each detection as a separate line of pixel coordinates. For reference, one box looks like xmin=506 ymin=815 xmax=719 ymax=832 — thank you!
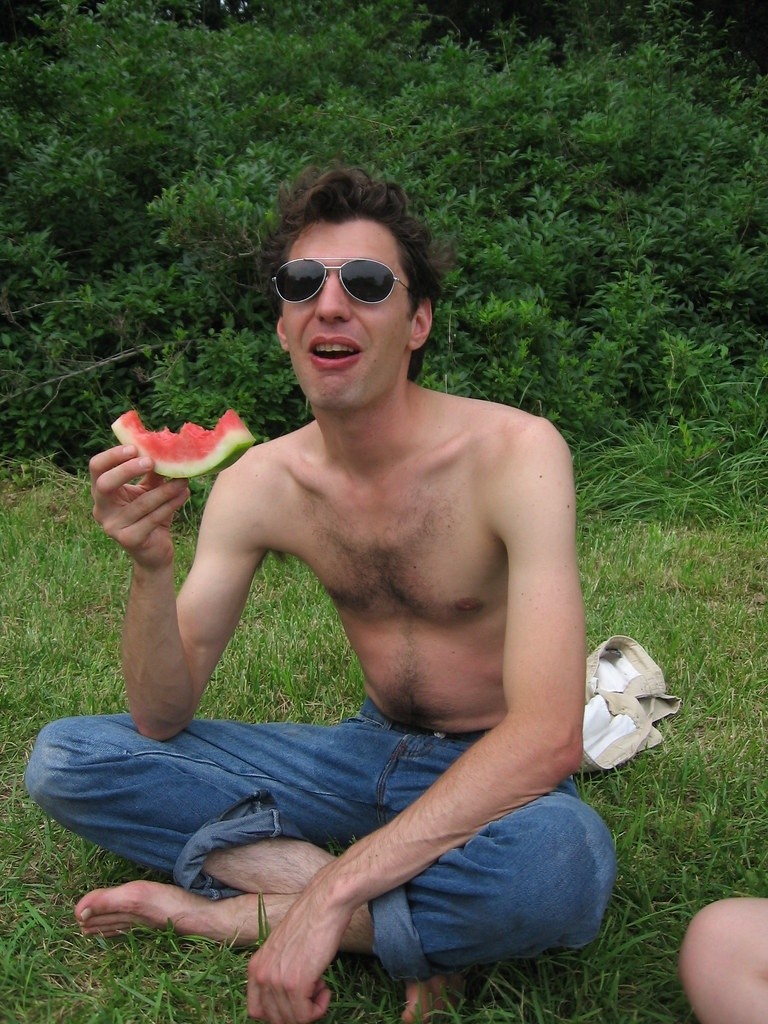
xmin=23 ymin=156 xmax=611 ymax=1023
xmin=678 ymin=897 xmax=768 ymax=1024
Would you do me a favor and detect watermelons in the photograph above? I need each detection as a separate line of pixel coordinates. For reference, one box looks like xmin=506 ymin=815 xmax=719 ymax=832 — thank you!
xmin=109 ymin=407 xmax=256 ymax=481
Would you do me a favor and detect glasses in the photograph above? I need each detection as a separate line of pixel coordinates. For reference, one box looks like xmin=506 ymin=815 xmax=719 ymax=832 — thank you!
xmin=271 ymin=258 xmax=421 ymax=304
